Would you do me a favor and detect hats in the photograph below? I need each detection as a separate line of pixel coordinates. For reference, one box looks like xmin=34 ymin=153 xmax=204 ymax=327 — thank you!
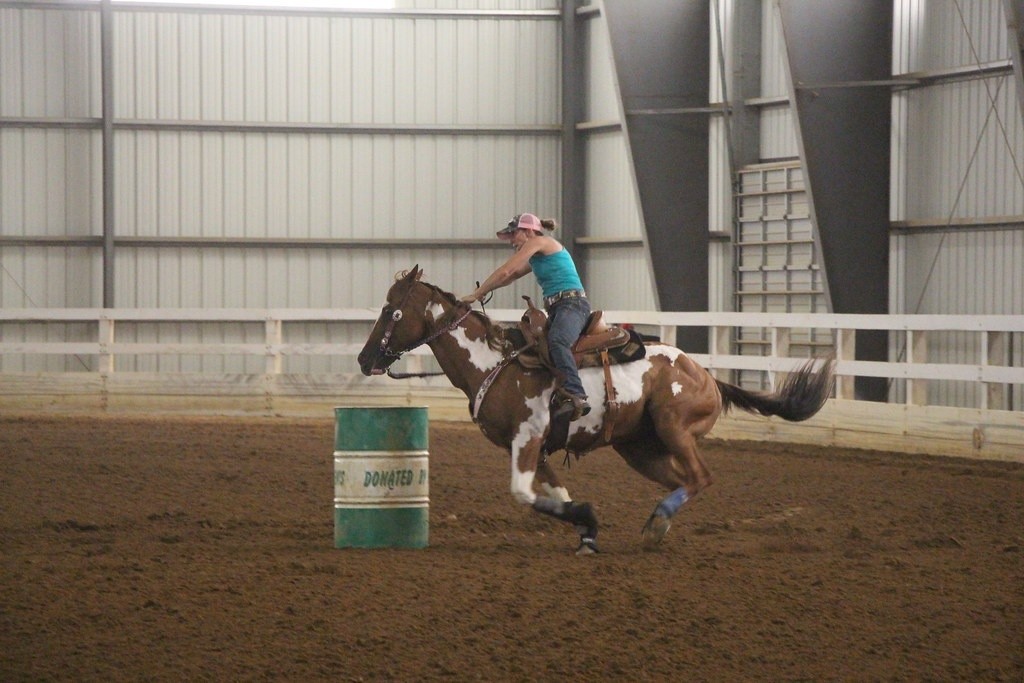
xmin=497 ymin=214 xmax=543 ymax=241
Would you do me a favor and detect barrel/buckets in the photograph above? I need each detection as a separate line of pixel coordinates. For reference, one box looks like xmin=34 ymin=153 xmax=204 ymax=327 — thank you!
xmin=333 ymin=404 xmax=430 ymax=550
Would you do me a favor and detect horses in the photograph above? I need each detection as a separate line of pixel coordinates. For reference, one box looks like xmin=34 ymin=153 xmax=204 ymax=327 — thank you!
xmin=357 ymin=263 xmax=839 ymax=557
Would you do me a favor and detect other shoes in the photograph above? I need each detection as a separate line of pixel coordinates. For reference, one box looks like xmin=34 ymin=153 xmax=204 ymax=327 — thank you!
xmin=554 ymin=397 xmax=591 ymax=420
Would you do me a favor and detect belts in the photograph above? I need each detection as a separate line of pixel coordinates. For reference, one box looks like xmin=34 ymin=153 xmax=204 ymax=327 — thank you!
xmin=546 ymin=289 xmax=586 ymax=310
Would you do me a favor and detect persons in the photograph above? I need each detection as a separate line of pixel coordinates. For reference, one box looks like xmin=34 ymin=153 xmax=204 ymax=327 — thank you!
xmin=461 ymin=212 xmax=592 ymax=421
